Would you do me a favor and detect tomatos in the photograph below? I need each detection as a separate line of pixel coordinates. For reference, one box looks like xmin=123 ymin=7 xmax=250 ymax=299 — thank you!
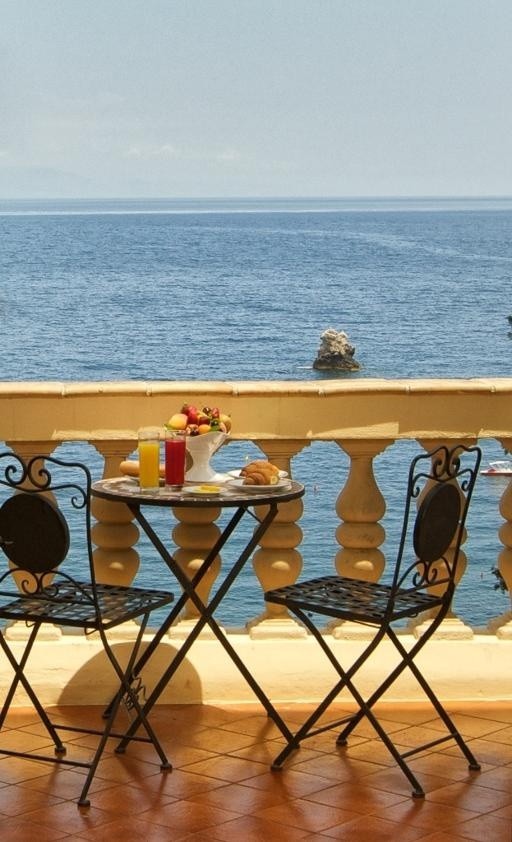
xmin=181 ymin=405 xmax=197 ymax=416
xmin=187 ymin=412 xmax=198 ymax=425
xmin=199 ymin=417 xmax=210 ymax=425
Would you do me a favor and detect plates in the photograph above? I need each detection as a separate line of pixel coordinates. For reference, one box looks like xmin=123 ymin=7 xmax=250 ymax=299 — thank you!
xmin=232 ymin=477 xmax=290 ymax=496
xmin=130 ymin=476 xmax=165 ymax=485
xmin=183 ymin=486 xmax=228 ymax=498
xmin=228 ymin=469 xmax=289 ymax=482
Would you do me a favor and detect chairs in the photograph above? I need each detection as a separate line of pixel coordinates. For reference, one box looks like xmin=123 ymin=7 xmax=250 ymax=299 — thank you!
xmin=0 ymin=452 xmax=173 ymax=807
xmin=263 ymin=445 xmax=482 ymax=797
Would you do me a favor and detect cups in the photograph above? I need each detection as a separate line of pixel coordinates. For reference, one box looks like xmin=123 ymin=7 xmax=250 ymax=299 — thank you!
xmin=137 ymin=432 xmax=161 ymax=497
xmin=165 ymin=430 xmax=185 ymax=493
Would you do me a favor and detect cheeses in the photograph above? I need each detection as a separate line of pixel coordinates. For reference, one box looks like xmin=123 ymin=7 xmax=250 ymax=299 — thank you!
xmin=197 ymin=486 xmax=219 ymax=493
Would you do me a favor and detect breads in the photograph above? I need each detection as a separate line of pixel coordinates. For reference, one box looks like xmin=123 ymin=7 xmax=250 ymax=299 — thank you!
xmin=242 ymin=470 xmax=277 ymax=484
xmin=239 ymin=461 xmax=279 ymax=476
xmin=120 ymin=461 xmax=166 ymax=477
